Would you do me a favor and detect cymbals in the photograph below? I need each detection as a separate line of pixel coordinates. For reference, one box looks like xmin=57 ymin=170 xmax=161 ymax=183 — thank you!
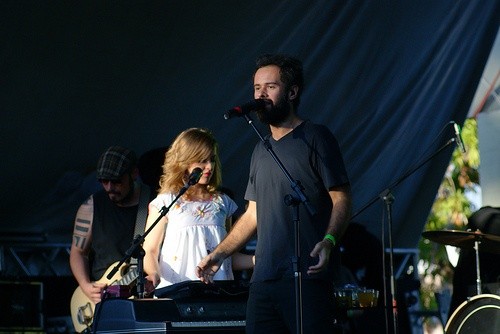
xmin=421 ymin=230 xmax=500 ymax=249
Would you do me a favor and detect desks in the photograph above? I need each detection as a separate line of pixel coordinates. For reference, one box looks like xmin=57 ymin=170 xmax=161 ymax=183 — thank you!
xmin=0 ymin=242 xmax=72 ymax=276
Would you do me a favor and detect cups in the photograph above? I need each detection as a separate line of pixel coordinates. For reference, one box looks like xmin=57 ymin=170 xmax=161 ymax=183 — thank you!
xmin=356 ymin=287 xmax=378 ymax=307
xmin=336 ymin=289 xmax=353 ymax=310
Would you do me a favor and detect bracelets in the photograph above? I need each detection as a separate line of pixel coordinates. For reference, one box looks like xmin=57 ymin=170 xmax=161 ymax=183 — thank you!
xmin=324 ymin=233 xmax=336 ymax=246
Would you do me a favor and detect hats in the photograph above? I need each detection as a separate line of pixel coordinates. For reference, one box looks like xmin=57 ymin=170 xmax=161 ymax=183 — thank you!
xmin=98 ymin=146 xmax=138 ymax=179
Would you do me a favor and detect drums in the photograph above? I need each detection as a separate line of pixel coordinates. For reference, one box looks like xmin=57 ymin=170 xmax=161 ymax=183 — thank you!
xmin=444 ymin=294 xmax=500 ymax=334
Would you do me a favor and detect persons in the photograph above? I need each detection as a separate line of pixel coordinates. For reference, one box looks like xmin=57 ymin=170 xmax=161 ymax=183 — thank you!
xmin=67 ymin=145 xmax=157 ymax=298
xmin=197 ymin=54 xmax=351 ymax=334
xmin=140 ymin=128 xmax=256 ymax=289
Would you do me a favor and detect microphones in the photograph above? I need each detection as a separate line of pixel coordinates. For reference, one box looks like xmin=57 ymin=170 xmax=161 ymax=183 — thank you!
xmin=223 ymin=99 xmax=265 ymax=120
xmin=453 ymin=124 xmax=466 ymax=153
xmin=189 ymin=167 xmax=202 ymax=185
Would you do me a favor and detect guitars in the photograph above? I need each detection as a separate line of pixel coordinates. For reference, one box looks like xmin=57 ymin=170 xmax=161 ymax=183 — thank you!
xmin=70 ymin=259 xmax=141 ymax=333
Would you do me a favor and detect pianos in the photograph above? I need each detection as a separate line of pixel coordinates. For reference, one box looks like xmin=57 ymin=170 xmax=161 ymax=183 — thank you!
xmin=91 ymin=280 xmax=339 ymax=334
